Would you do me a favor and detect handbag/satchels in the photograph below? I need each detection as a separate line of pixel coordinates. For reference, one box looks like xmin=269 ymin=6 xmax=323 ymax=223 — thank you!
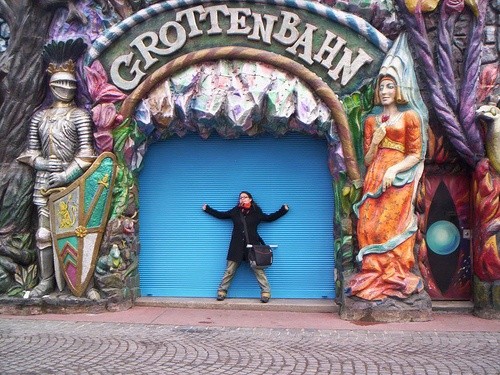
xmin=248 ymin=243 xmax=274 ymax=269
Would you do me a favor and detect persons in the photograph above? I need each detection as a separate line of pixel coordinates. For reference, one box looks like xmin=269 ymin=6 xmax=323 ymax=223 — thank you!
xmin=203 ymin=190 xmax=289 ymax=303
xmin=358 ymin=74 xmax=424 ymax=275
xmin=18 ymin=72 xmax=101 ymax=299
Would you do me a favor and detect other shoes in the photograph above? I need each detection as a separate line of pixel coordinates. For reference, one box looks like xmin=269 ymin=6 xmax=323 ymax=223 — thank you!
xmin=217 ymin=289 xmax=227 ymax=301
xmin=261 ymin=292 xmax=272 ymax=302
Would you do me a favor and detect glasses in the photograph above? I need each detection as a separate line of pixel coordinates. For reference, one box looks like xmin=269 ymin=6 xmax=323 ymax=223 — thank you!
xmin=239 ymin=195 xmax=251 ymax=200
